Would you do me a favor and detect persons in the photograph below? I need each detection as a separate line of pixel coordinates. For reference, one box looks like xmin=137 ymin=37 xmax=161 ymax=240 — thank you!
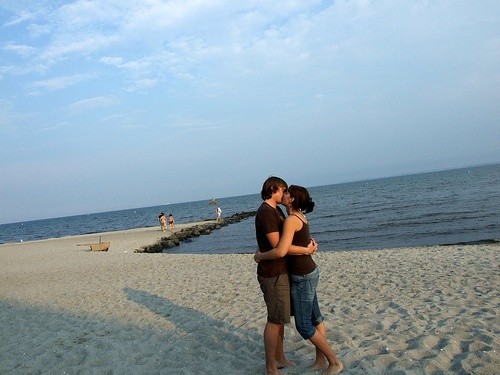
xmin=255 ymin=177 xmax=319 ymax=375
xmin=158 ymin=212 xmax=174 ymax=232
xmin=254 ymin=185 xmax=343 ymax=375
xmin=216 ymin=206 xmax=223 ymax=223
